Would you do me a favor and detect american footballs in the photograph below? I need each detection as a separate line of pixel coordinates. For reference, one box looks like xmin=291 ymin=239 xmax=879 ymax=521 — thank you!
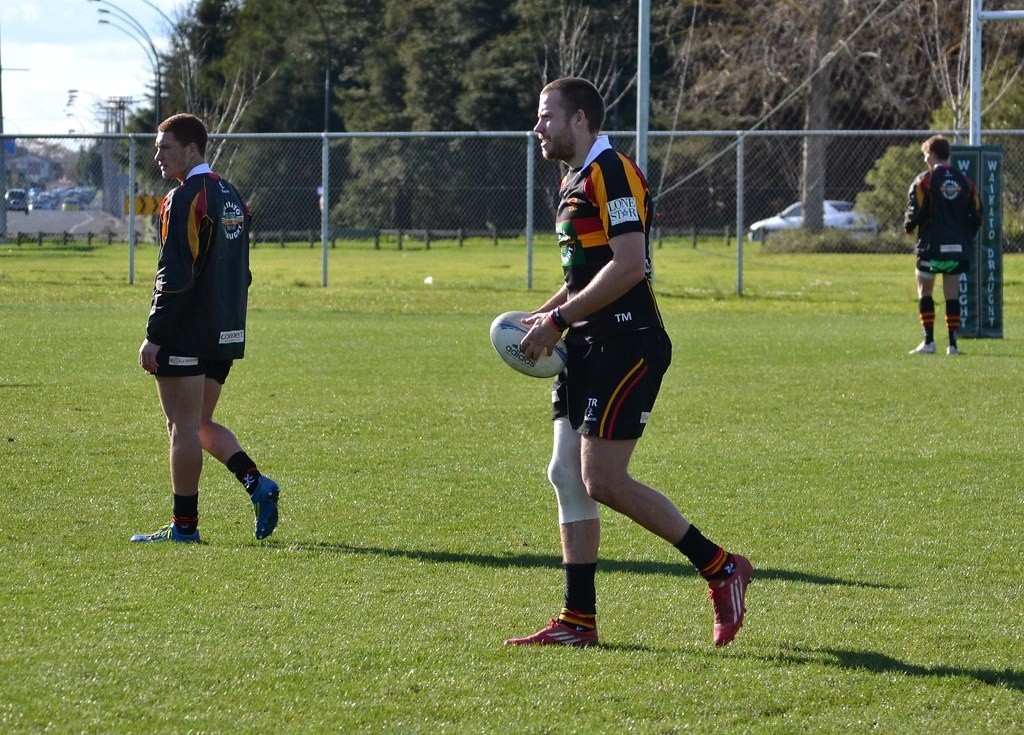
xmin=490 ymin=310 xmax=568 ymax=378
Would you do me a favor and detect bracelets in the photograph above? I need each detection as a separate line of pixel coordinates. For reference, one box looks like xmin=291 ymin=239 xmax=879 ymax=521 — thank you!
xmin=548 ymin=307 xmax=570 ymax=332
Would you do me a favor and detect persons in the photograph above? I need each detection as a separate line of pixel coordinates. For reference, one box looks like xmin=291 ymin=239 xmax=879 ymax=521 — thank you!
xmin=506 ymin=76 xmax=753 ymax=647
xmin=904 ymin=134 xmax=983 ymax=355
xmin=126 ymin=114 xmax=281 ymax=544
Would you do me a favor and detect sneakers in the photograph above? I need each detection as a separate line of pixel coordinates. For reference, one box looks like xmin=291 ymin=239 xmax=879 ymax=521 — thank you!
xmin=709 ymin=554 xmax=754 ymax=648
xmin=908 ymin=341 xmax=936 ymax=354
xmin=947 ymin=346 xmax=960 ymax=355
xmin=253 ymin=477 xmax=280 ymax=539
xmin=504 ymin=618 xmax=598 ymax=647
xmin=130 ymin=523 xmax=200 ymax=545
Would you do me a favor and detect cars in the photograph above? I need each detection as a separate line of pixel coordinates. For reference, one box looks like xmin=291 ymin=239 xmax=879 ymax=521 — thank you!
xmin=4 ymin=187 xmax=97 ymax=211
xmin=748 ymin=200 xmax=879 ymax=242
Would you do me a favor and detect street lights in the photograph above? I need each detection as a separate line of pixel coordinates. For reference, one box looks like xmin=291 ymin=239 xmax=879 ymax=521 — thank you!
xmin=81 ymin=0 xmax=201 ymax=128
